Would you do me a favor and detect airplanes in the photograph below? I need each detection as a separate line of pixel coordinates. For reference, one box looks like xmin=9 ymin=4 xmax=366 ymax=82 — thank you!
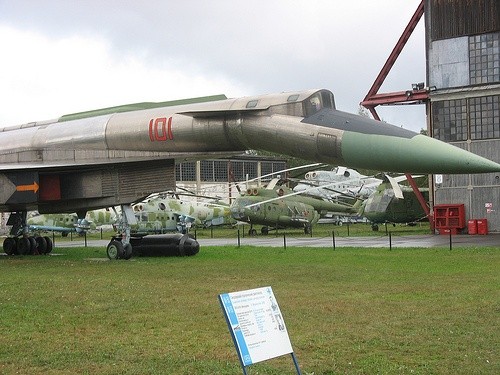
xmin=0 ymin=89 xmax=500 ymax=259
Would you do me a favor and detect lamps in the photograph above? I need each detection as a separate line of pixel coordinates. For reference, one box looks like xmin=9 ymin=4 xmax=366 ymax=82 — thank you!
xmin=406 ymin=90 xmax=412 ymax=96
xmin=417 ymin=82 xmax=425 ymax=90
xmin=430 ymin=85 xmax=438 ymax=91
xmin=412 ymin=83 xmax=417 ymax=90
